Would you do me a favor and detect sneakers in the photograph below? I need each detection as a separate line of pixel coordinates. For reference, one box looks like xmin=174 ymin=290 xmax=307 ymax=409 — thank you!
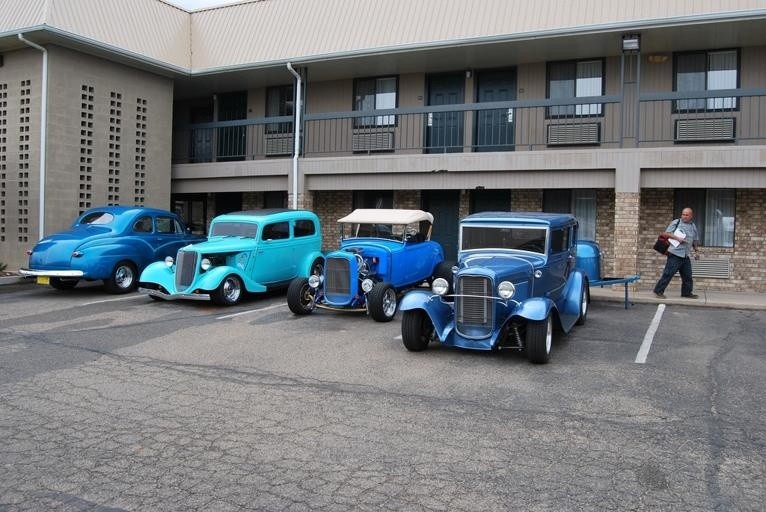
xmin=682 ymin=293 xmax=698 ymax=298
xmin=654 ymin=289 xmax=665 ymax=298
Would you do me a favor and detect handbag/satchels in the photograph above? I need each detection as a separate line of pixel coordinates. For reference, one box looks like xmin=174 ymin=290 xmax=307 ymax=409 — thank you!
xmin=653 ymin=236 xmax=670 ymax=254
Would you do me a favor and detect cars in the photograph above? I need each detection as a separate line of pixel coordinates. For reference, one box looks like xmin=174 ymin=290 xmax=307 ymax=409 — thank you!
xmin=17 ymin=204 xmax=208 ymax=293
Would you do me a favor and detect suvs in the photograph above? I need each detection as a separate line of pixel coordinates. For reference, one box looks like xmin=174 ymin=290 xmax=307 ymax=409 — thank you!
xmin=288 ymin=206 xmax=457 ymax=324
xmin=138 ymin=207 xmax=328 ymax=306
xmin=400 ymin=210 xmax=590 ymax=363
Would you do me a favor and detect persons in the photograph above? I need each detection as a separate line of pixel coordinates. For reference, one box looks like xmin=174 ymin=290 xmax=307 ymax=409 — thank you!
xmin=652 ymin=207 xmax=701 ymax=299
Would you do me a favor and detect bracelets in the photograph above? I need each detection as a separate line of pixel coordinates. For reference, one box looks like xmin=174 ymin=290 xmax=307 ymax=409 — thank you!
xmin=694 ymin=249 xmax=698 ymax=252
